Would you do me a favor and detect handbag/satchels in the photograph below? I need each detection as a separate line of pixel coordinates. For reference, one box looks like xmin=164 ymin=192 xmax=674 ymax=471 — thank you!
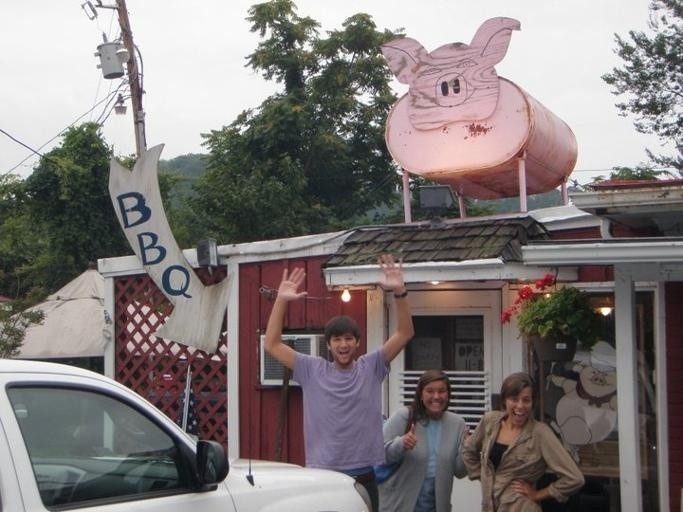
xmin=372 ymin=458 xmax=403 ymax=485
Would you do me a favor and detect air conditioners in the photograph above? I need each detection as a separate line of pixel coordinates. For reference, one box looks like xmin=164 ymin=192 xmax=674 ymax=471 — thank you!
xmin=260 ymin=334 xmax=331 ymax=388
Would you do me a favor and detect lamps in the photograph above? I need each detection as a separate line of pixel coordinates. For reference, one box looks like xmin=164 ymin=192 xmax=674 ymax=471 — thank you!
xmin=341 ymin=285 xmax=350 ymax=302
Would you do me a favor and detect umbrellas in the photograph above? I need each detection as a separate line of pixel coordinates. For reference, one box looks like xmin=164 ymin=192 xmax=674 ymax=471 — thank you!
xmin=0 ymin=261 xmax=223 ymax=373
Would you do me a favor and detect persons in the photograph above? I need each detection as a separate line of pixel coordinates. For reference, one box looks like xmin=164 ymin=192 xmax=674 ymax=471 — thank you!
xmin=262 ymin=253 xmax=416 ymax=511
xmin=462 ymin=373 xmax=586 ymax=512
xmin=373 ymin=368 xmax=471 ymax=512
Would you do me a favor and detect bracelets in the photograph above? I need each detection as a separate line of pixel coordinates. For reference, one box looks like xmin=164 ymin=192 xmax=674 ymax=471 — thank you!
xmin=393 ymin=290 xmax=408 ymax=298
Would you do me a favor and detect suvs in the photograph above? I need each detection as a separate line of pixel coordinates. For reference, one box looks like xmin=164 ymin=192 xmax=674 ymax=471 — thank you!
xmin=0 ymin=356 xmax=378 ymax=512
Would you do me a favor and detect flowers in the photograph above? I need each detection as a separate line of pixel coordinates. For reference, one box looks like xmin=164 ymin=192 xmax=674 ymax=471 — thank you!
xmin=498 ymin=271 xmax=598 ymax=348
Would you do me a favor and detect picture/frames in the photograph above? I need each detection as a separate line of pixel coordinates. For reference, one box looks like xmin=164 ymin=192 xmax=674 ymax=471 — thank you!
xmin=539 ymin=304 xmax=650 ymax=479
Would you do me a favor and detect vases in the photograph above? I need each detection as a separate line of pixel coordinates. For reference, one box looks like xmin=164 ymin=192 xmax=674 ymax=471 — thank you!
xmin=531 ymin=334 xmax=575 ymax=362
